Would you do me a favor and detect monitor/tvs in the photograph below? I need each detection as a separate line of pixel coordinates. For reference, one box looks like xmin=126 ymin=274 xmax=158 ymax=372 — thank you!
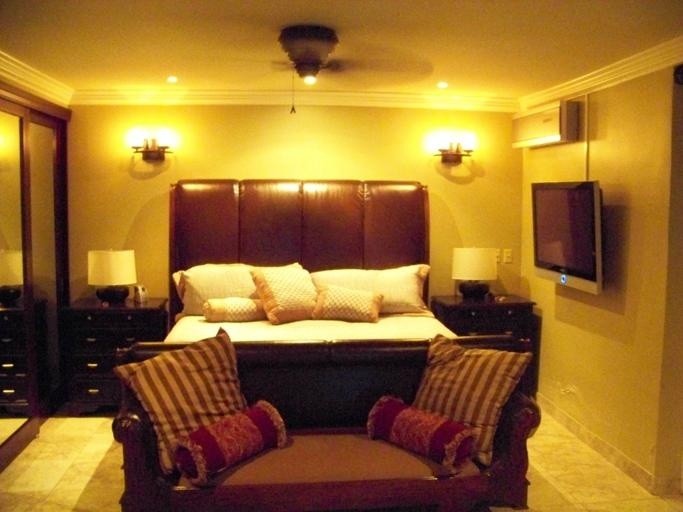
xmin=531 ymin=179 xmax=604 ymax=296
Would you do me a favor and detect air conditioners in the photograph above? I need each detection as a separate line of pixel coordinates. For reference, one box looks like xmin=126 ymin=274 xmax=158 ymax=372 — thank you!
xmin=509 ymin=100 xmax=582 ymax=151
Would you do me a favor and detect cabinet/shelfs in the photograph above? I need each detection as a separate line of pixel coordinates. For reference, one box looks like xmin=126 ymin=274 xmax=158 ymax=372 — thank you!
xmin=0 ymin=302 xmax=45 ymax=417
xmin=436 ymin=291 xmax=542 ymax=403
xmin=67 ymin=296 xmax=165 ymax=415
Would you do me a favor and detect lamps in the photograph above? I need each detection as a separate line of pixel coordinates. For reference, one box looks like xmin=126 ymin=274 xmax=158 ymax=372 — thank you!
xmin=0 ymin=248 xmax=23 ymax=308
xmin=449 ymin=246 xmax=503 ymax=297
xmin=428 ymin=129 xmax=478 ymax=165
xmin=88 ymin=247 xmax=137 ymax=302
xmin=129 ymin=125 xmax=172 ymax=165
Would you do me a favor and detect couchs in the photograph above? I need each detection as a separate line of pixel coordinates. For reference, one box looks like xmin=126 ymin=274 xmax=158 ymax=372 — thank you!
xmin=109 ymin=339 xmax=540 ymax=512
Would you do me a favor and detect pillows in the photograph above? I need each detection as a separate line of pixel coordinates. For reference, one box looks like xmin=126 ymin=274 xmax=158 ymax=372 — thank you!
xmin=167 ymin=261 xmax=304 ymax=317
xmin=311 ymin=281 xmax=381 ymax=320
xmin=170 ymin=401 xmax=285 ymax=485
xmin=364 ymin=394 xmax=473 ymax=479
xmin=310 ymin=264 xmax=432 ymax=313
xmin=413 ymin=333 xmax=532 ymax=465
xmin=250 ymin=261 xmax=316 ymax=323
xmin=112 ymin=328 xmax=246 ymax=473
xmin=202 ymin=297 xmax=268 ymax=321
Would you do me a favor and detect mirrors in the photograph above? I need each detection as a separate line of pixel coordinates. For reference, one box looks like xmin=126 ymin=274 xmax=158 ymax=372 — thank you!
xmin=0 ymin=99 xmax=36 ymax=473
xmin=24 ymin=121 xmax=81 ymax=412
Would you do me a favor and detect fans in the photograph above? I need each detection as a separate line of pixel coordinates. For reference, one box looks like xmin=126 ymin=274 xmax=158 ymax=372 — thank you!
xmin=270 ymin=24 xmax=434 ymax=86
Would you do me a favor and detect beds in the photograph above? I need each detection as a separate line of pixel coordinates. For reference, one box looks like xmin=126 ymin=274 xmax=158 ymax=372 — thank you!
xmin=155 ymin=176 xmax=463 ymax=340
